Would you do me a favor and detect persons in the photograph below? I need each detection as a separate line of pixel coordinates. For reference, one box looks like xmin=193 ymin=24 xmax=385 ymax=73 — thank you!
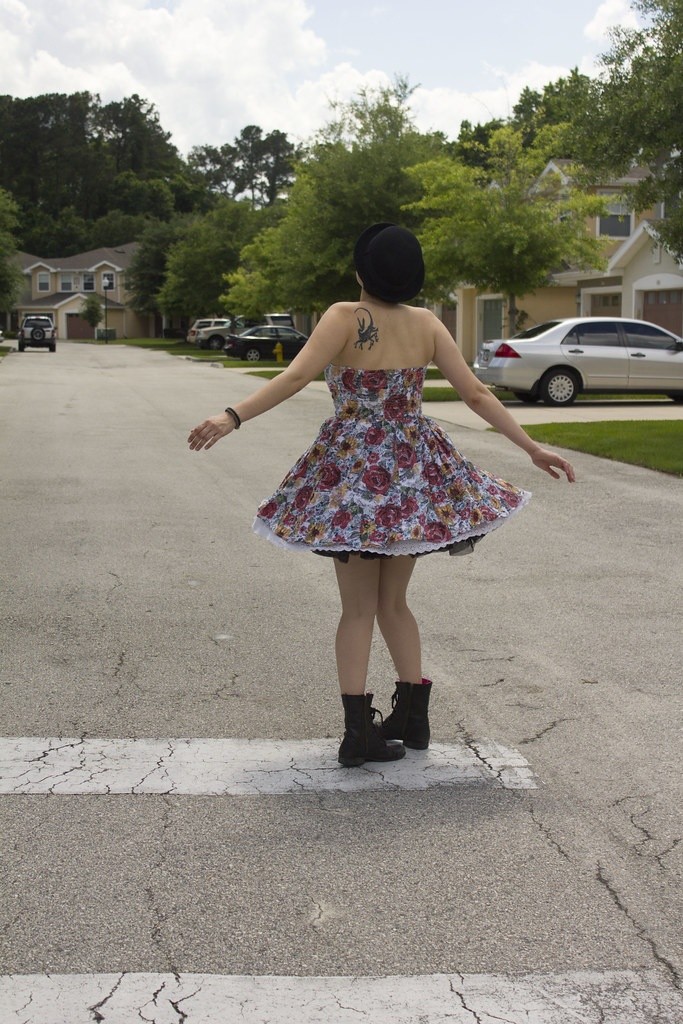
xmin=188 ymin=222 xmax=574 ymax=766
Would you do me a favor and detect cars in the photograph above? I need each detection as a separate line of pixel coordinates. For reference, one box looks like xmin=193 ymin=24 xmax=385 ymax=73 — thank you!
xmin=223 ymin=325 xmax=309 ymax=360
xmin=470 ymin=316 xmax=683 ymax=410
xmin=186 ymin=318 xmax=231 ymax=346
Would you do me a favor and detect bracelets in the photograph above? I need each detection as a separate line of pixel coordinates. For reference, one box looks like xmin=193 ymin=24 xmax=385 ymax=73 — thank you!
xmin=225 ymin=407 xmax=241 ymax=429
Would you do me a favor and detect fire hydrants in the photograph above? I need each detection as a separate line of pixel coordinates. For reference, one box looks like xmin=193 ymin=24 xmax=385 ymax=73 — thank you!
xmin=272 ymin=341 xmax=286 ymax=363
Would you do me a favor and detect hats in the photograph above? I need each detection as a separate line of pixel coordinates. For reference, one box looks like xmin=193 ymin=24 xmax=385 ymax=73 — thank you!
xmin=352 ymin=222 xmax=425 ymax=303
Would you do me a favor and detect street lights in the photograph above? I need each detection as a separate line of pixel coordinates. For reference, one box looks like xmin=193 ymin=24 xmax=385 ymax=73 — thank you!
xmin=102 ymin=276 xmax=111 ymax=344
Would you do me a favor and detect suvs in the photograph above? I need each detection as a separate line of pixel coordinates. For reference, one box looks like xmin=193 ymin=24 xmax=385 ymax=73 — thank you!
xmin=18 ymin=316 xmax=57 ymax=352
xmin=195 ymin=313 xmax=296 ymax=351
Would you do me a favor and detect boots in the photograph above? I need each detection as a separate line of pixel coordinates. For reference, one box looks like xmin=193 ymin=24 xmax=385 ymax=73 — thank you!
xmin=337 ymin=693 xmax=406 ymax=766
xmin=371 ymin=678 xmax=433 ymax=750
